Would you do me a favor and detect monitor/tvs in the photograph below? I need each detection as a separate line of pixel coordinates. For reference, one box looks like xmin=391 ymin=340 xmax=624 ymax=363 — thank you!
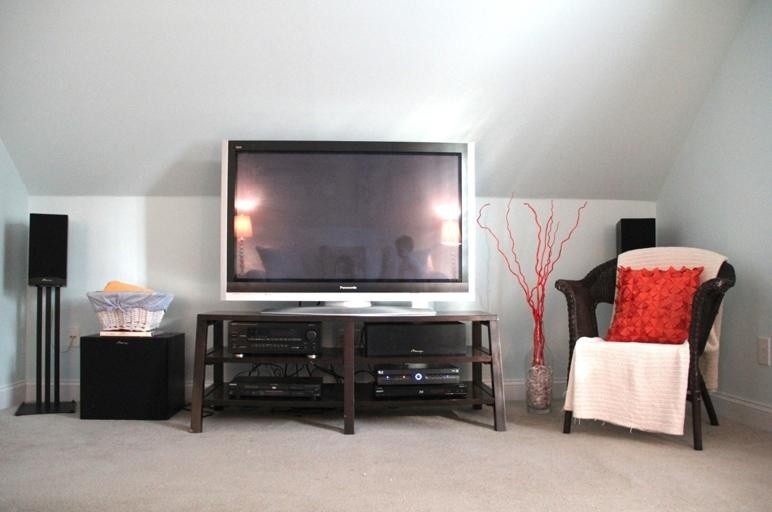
xmin=218 ymin=139 xmax=478 ymax=315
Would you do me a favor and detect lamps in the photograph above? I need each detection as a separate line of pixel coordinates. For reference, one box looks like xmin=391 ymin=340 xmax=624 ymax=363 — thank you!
xmin=235 ymin=214 xmax=254 ymax=277
xmin=440 ymin=220 xmax=465 ymax=278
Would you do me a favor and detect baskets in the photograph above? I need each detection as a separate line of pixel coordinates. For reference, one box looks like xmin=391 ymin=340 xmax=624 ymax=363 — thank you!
xmin=87 ymin=290 xmax=174 ymax=332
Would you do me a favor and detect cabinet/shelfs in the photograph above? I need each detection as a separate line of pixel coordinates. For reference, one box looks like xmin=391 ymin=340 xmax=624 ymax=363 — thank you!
xmin=190 ymin=309 xmax=508 ymax=434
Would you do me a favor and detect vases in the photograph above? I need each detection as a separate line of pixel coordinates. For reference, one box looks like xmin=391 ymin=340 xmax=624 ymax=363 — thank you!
xmin=522 ymin=361 xmax=554 ymax=411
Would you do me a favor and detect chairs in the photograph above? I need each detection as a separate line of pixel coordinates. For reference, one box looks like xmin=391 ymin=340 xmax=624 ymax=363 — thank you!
xmin=554 ymin=246 xmax=737 ymax=451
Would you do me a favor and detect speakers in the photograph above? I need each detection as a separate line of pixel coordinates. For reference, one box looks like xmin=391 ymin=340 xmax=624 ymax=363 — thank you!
xmin=79 ymin=333 xmax=187 ymax=420
xmin=27 ymin=210 xmax=70 ymax=289
xmin=364 ymin=320 xmax=468 ymax=357
xmin=616 ymin=216 xmax=656 ymax=255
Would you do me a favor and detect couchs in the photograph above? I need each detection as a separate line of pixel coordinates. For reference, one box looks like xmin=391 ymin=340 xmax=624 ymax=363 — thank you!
xmin=246 ymin=268 xmax=446 ymax=280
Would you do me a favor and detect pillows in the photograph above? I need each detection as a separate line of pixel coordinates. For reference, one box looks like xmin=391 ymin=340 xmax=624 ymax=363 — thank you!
xmin=604 ymin=263 xmax=705 ymax=344
xmin=321 ymin=247 xmax=367 ymax=278
xmin=255 ymin=243 xmax=309 ymax=277
xmin=382 ymin=249 xmax=433 ymax=278
